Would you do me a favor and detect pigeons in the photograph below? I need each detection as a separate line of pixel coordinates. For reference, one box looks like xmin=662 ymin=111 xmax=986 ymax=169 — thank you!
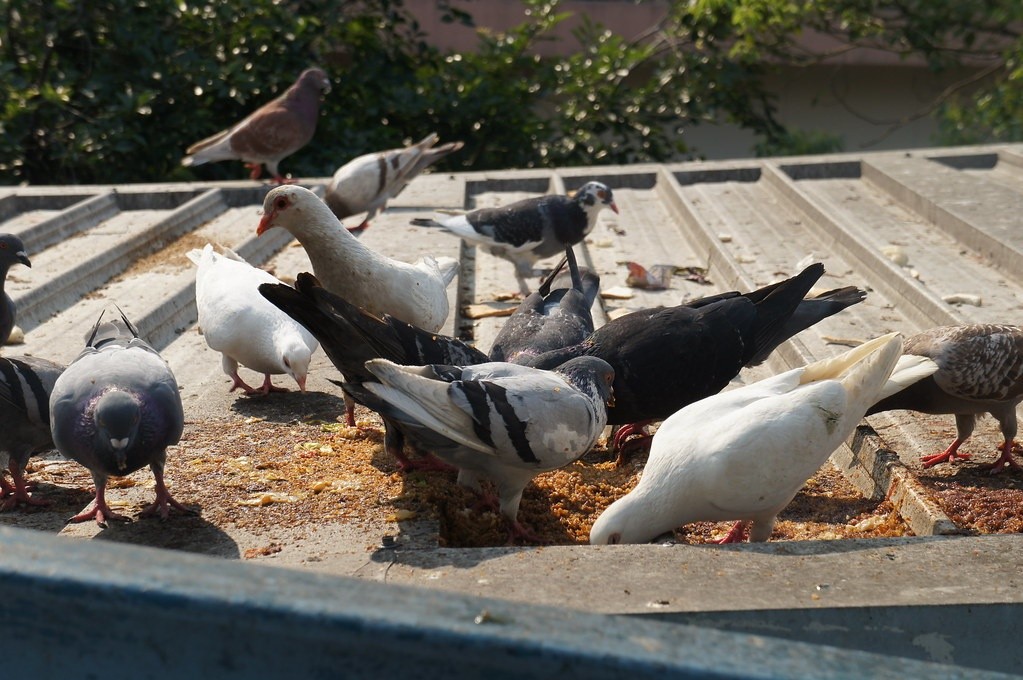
xmin=1 ymin=234 xmax=199 ymax=524
xmin=188 ymin=134 xmax=1023 ymax=546
xmin=181 ymin=68 xmax=332 ymax=182
